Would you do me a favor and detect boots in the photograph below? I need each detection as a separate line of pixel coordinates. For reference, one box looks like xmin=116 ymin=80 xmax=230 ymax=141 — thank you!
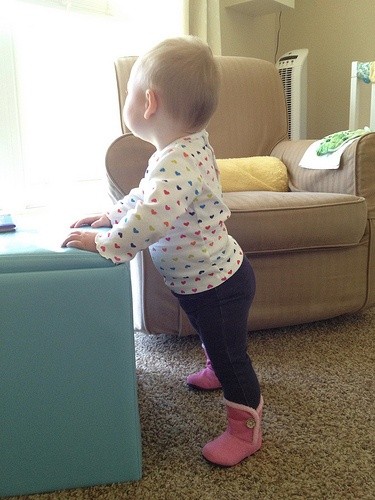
xmin=187 ymin=343 xmax=223 ymax=390
xmin=202 ymin=394 xmax=264 ymax=467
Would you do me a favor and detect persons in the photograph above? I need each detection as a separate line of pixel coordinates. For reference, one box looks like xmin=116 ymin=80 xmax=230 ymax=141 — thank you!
xmin=61 ymin=35 xmax=264 ymax=466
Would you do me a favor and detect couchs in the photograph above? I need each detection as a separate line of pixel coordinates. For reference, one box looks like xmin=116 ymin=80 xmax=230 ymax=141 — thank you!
xmin=102 ymin=56 xmax=375 ymax=337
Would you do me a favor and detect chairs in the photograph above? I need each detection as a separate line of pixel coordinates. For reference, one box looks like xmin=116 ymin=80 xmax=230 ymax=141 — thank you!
xmin=348 ymin=61 xmax=375 ymax=131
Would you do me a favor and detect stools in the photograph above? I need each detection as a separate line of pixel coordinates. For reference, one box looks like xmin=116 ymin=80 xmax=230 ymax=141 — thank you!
xmin=0 ymin=216 xmax=143 ymax=497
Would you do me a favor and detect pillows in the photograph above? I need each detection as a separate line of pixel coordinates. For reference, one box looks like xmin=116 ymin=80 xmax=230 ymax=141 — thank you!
xmin=216 ymin=155 xmax=289 ymax=192
